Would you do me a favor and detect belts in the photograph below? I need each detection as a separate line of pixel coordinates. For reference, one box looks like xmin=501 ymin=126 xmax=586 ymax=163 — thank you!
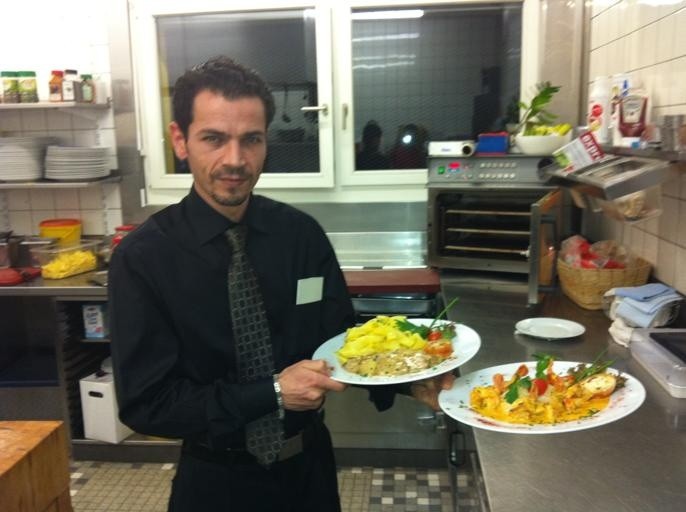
xmin=182 ymin=409 xmax=327 ymax=470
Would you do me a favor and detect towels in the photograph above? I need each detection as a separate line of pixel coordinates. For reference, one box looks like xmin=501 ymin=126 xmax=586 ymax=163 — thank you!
xmin=613 ymin=281 xmax=685 ymax=330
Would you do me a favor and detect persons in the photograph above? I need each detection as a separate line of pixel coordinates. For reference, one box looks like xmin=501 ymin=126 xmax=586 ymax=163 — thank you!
xmin=107 ymin=56 xmax=458 ymax=511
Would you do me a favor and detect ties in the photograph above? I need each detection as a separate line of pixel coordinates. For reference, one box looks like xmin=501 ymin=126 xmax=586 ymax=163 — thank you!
xmin=225 ymin=225 xmax=286 ymax=471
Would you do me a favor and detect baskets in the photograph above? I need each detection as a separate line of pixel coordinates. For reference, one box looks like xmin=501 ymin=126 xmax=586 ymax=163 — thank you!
xmin=552 ymin=250 xmax=653 ymax=314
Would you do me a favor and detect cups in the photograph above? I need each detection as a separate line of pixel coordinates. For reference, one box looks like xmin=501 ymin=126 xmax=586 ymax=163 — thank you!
xmin=655 ymin=115 xmax=685 ymax=152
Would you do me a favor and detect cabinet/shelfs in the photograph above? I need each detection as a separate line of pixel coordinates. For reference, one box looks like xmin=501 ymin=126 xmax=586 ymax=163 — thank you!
xmin=0 ymin=418 xmax=76 ymax=511
xmin=125 ymin=0 xmax=546 ymax=209
xmin=0 ymin=101 xmax=122 ymax=190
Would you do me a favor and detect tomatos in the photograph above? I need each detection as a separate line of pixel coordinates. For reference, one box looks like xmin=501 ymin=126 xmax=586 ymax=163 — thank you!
xmin=428 ymin=331 xmax=441 ymax=341
xmin=530 ymin=378 xmax=547 ymax=396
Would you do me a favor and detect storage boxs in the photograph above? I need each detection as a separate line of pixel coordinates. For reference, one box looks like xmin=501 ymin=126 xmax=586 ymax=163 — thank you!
xmin=79 ymin=371 xmax=135 ymax=445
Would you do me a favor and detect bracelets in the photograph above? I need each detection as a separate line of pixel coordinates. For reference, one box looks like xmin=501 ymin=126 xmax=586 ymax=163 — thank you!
xmin=272 ymin=374 xmax=289 ymax=422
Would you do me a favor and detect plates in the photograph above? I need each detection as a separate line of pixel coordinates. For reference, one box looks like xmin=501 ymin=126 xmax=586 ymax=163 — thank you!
xmin=515 ymin=316 xmax=585 ymax=342
xmin=439 ymin=359 xmax=648 ymax=435
xmin=311 ymin=316 xmax=480 ymax=386
xmin=0 ymin=134 xmax=111 ymax=181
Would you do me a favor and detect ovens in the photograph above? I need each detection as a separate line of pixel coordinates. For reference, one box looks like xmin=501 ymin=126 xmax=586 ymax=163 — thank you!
xmin=426 ymin=156 xmax=571 ymax=307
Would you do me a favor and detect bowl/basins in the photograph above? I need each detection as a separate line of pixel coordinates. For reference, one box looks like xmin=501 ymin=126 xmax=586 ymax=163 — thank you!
xmin=510 ymin=135 xmax=565 ymax=158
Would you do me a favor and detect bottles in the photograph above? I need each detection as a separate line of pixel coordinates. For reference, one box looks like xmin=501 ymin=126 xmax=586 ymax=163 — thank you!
xmin=112 ymin=226 xmax=135 ymax=246
xmin=0 ymin=70 xmax=96 ymax=105
xmin=587 ymin=74 xmax=650 ymax=149
